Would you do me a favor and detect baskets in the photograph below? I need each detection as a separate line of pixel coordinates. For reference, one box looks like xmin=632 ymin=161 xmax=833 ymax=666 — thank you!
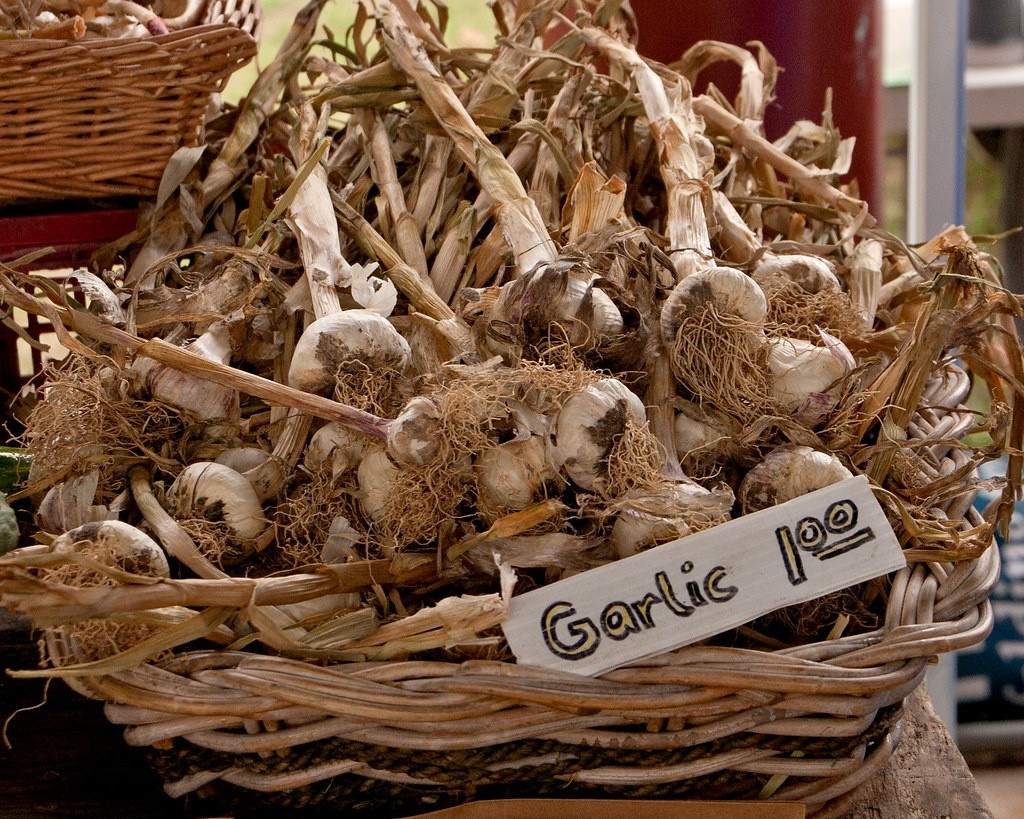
xmin=38 ymin=382 xmax=992 ymax=811
xmin=0 ymin=1 xmax=251 ymax=212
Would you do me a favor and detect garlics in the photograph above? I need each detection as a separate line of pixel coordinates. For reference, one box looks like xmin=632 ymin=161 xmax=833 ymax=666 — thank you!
xmin=45 ymin=254 xmax=860 ymax=580
